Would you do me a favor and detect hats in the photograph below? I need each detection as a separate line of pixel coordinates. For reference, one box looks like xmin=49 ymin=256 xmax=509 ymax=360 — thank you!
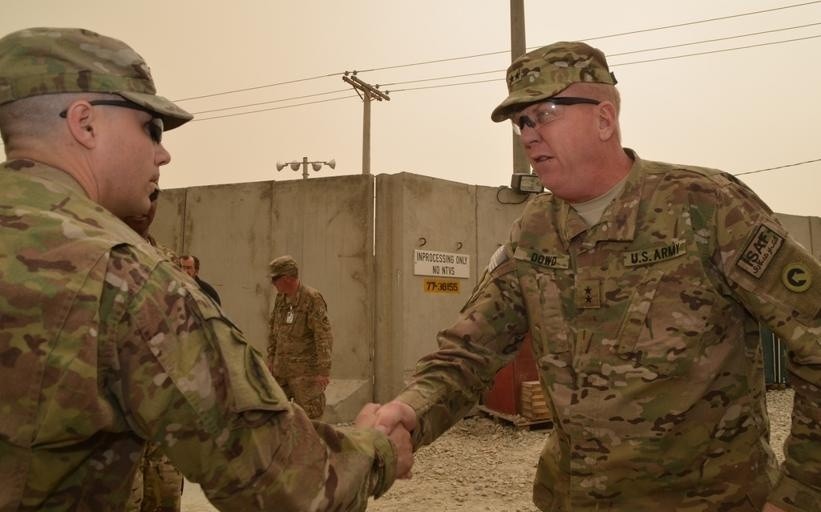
xmin=264 ymin=255 xmax=298 ymax=278
xmin=491 ymin=41 xmax=617 ymax=122
xmin=0 ymin=26 xmax=195 ymax=132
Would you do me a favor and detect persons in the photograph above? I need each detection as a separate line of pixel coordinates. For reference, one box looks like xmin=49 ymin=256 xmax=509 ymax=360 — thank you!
xmin=376 ymin=40 xmax=821 ymax=510
xmin=177 ymin=254 xmax=221 ymax=309
xmin=1 ymin=26 xmax=417 ymax=512
xmin=261 ymin=254 xmax=338 ymax=426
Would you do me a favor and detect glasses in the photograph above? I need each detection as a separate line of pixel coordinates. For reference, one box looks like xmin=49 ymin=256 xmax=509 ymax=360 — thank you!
xmin=59 ymin=98 xmax=164 ymax=145
xmin=510 ymin=96 xmax=600 ymax=136
